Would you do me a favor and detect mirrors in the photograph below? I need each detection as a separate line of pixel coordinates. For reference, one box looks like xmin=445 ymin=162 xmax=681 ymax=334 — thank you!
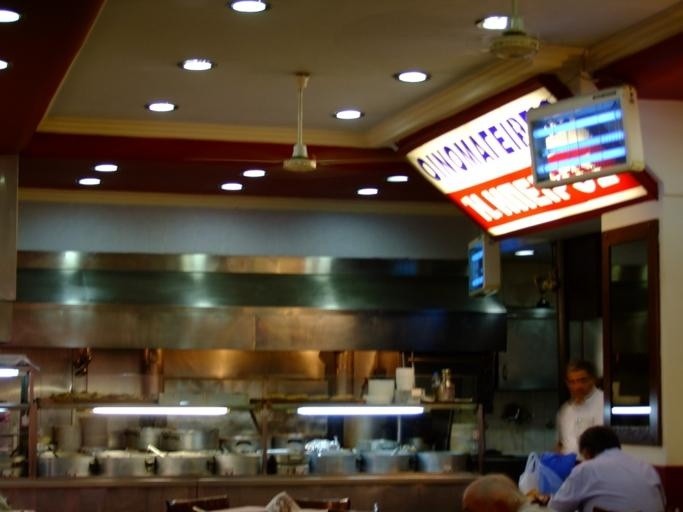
xmin=598 ymin=217 xmax=663 ymax=448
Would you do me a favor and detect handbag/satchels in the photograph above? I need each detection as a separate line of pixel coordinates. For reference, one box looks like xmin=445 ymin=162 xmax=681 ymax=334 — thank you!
xmin=540 ymin=450 xmax=577 ymax=496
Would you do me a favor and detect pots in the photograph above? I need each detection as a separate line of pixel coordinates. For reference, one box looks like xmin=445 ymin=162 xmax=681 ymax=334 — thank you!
xmin=35 ymin=414 xmax=470 ymax=477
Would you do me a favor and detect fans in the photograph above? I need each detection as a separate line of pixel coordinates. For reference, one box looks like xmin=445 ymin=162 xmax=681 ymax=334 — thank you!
xmin=185 ymin=74 xmax=403 ymax=176
xmin=369 ymin=0 xmax=591 ymax=73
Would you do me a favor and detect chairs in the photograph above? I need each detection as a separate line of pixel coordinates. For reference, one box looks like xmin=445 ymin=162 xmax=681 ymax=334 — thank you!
xmin=161 ymin=490 xmax=230 ymax=511
xmin=289 ymin=494 xmax=352 ymax=511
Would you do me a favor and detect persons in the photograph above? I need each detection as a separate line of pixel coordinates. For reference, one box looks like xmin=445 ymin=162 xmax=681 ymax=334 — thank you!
xmin=461 ymin=358 xmax=668 ymax=512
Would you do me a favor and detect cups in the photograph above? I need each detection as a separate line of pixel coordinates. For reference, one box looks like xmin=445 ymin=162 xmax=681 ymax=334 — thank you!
xmin=394 ymin=367 xmax=417 ymax=390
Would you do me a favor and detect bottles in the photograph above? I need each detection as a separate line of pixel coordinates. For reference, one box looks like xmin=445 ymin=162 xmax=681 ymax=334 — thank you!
xmin=431 ymin=369 xmax=455 ymax=403
